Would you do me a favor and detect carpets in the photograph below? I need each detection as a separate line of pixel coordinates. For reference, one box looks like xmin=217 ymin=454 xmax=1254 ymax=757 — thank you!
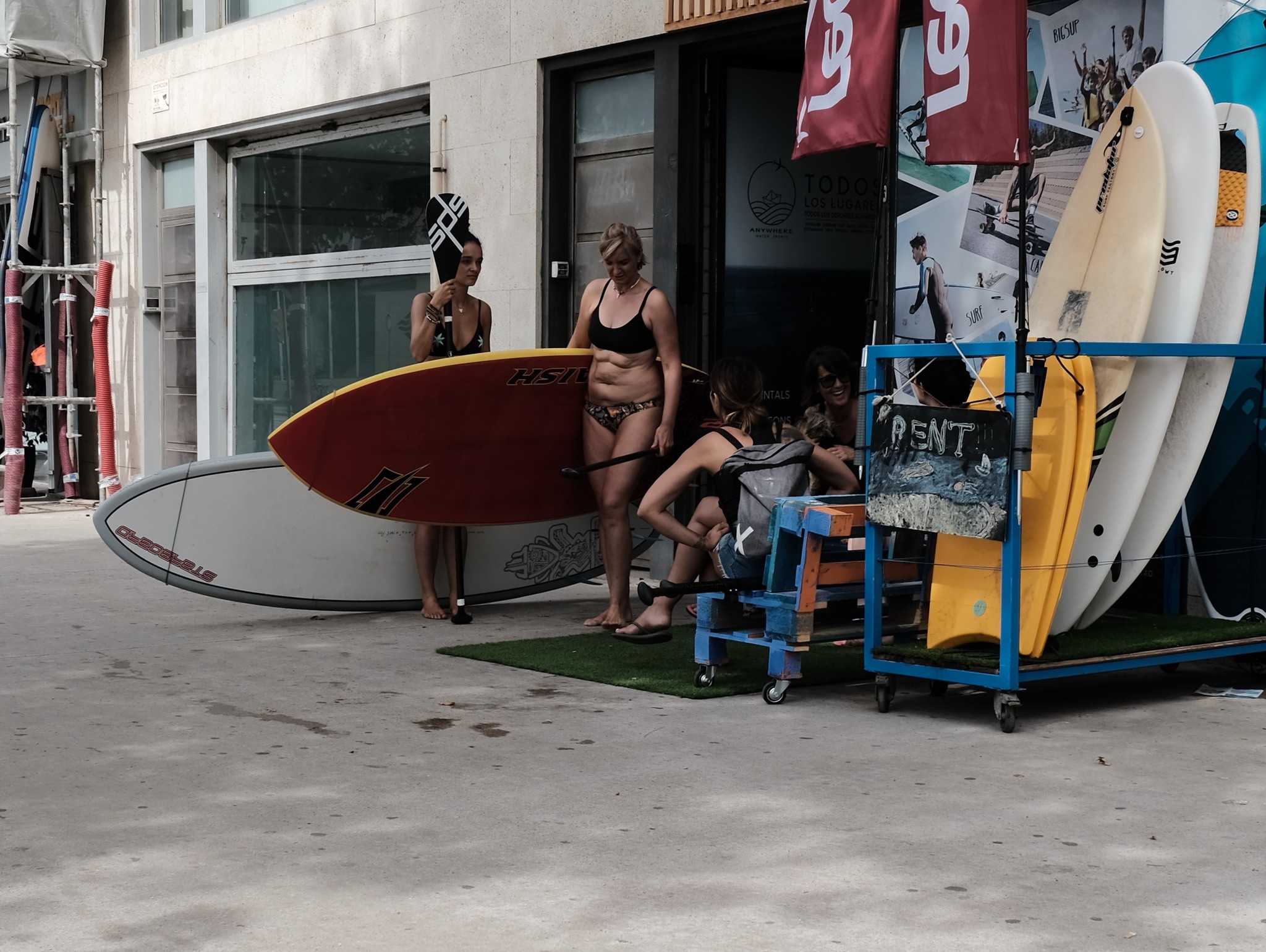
xmin=436 ymin=623 xmax=877 ymax=698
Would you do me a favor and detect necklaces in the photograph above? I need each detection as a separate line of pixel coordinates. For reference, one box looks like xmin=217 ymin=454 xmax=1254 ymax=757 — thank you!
xmin=452 ymin=295 xmax=467 ymax=313
xmin=613 ymin=274 xmax=641 ymax=299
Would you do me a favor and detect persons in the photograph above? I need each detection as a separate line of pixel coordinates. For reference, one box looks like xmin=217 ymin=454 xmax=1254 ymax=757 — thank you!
xmin=981 ymin=132 xmax=1055 ymax=235
xmin=908 ymin=357 xmax=973 ymax=407
xmin=688 ymin=347 xmax=866 ymax=620
xmin=1073 ymin=0 xmax=1163 ymax=133
xmin=610 ymin=357 xmax=861 ymax=654
xmin=907 ymin=234 xmax=954 ymax=345
xmin=410 ymin=231 xmax=492 ymax=621
xmin=565 ymin=221 xmax=682 ymax=628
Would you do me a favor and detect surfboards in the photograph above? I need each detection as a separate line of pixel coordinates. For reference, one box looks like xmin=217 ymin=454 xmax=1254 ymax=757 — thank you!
xmin=267 ymin=351 xmax=711 ymax=527
xmin=952 ymin=62 xmax=1259 ymax=637
xmin=0 ymin=104 xmax=61 ymax=497
xmin=94 ymin=453 xmax=663 ymax=611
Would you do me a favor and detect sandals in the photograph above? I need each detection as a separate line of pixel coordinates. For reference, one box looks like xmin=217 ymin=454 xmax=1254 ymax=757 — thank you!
xmin=611 ymin=621 xmax=673 ymax=644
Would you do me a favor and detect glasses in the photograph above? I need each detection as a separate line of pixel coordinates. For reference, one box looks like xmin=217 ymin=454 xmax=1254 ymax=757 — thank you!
xmin=818 ymin=372 xmax=850 ymax=388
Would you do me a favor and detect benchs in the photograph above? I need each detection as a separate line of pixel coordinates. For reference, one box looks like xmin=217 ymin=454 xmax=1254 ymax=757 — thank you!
xmin=694 ymin=493 xmax=937 ymax=704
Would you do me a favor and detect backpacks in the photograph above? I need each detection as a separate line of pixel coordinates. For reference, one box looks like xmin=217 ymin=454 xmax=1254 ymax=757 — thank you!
xmin=718 ymin=438 xmax=812 ymax=561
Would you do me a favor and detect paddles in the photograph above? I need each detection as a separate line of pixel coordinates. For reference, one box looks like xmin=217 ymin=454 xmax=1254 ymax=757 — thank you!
xmin=637 ymin=577 xmax=766 ymax=607
xmin=425 ymin=193 xmax=471 ymax=620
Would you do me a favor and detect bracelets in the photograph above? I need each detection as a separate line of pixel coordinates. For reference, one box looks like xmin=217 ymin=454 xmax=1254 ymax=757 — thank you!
xmin=425 ymin=303 xmax=443 ymax=324
